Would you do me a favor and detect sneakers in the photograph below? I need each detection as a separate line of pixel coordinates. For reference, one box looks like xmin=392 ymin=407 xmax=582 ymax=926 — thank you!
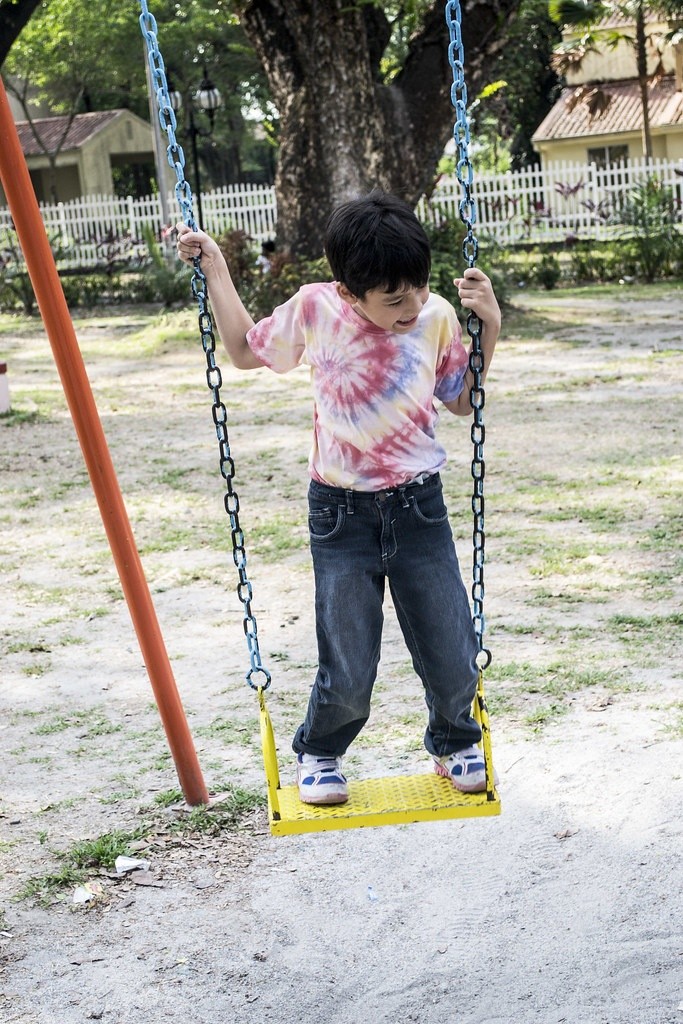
xmin=433 ymin=741 xmax=499 ymax=792
xmin=293 ymin=752 xmax=350 ymax=804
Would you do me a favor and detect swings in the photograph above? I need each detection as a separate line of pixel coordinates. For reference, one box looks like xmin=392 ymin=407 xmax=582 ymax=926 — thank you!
xmin=134 ymin=0 xmax=504 ymax=839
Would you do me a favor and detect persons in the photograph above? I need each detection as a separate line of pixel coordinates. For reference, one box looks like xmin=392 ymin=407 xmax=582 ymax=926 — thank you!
xmin=175 ymin=191 xmax=502 ymax=806
